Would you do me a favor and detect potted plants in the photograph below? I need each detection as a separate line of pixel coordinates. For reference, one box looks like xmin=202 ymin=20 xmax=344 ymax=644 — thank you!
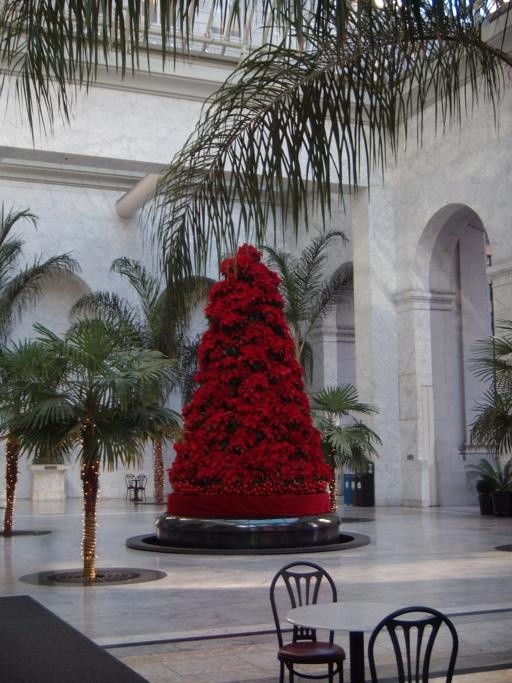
xmin=464 ymin=455 xmax=511 ymax=517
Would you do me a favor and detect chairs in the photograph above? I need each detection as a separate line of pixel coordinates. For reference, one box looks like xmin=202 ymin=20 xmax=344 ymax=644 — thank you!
xmin=125 ymin=473 xmax=148 ymax=503
xmin=367 ymin=606 xmax=460 ymax=682
xmin=270 ymin=560 xmax=346 ymax=682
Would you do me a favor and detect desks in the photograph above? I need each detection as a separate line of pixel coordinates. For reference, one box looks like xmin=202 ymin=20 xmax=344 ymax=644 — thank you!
xmin=285 ymin=600 xmax=427 ymax=682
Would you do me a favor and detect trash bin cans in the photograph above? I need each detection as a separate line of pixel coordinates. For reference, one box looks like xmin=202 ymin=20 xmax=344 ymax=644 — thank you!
xmin=343 ymin=460 xmax=374 ymax=507
